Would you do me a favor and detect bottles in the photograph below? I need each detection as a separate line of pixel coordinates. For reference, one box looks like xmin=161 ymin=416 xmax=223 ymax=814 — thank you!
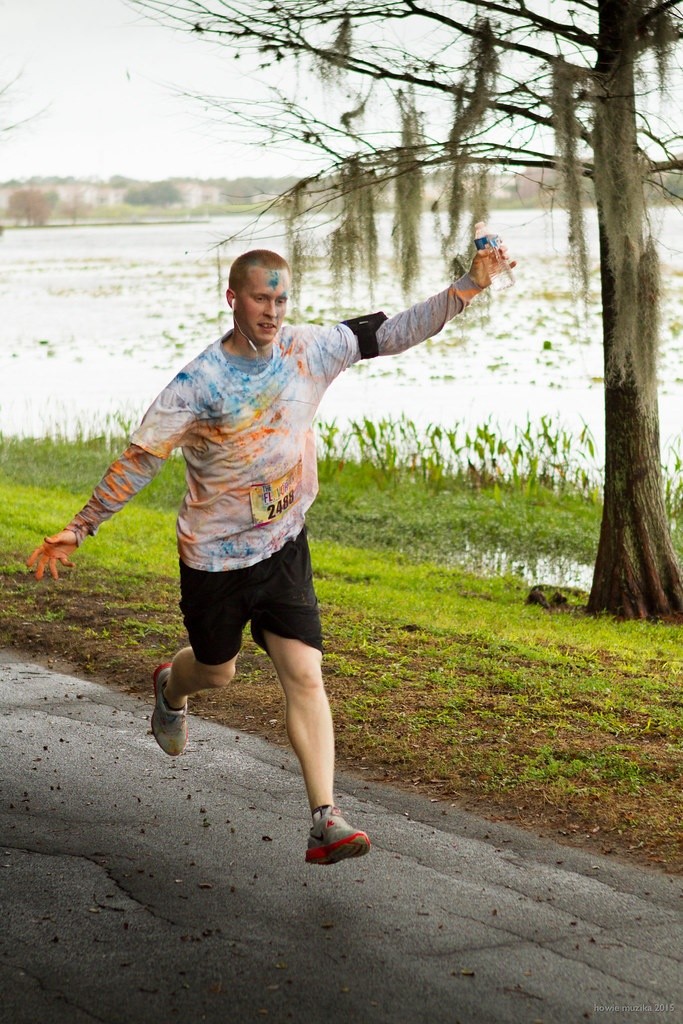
xmin=474 ymin=222 xmax=516 ymax=293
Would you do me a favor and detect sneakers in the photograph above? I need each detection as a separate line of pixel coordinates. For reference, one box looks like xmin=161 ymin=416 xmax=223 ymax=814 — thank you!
xmin=304 ymin=805 xmax=369 ymax=865
xmin=150 ymin=662 xmax=188 ymax=756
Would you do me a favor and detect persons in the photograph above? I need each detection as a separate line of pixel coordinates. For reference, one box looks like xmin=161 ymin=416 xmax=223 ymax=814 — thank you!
xmin=23 ymin=238 xmax=517 ymax=863
